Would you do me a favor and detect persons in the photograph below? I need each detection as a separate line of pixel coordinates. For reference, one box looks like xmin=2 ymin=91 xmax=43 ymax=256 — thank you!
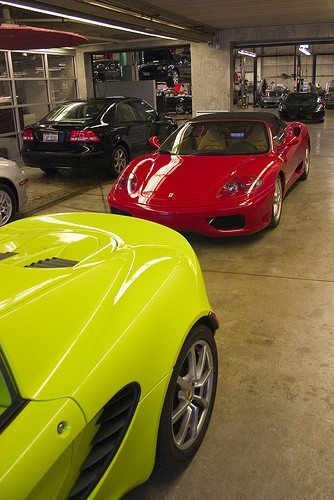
xmin=244 ymin=80 xmax=252 ymax=93
xmin=258 ymin=79 xmax=274 ymax=97
xmin=301 ymin=79 xmax=324 ymax=96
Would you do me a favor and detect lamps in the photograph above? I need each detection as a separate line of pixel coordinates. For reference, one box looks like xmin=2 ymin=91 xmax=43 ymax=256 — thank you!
xmin=299 ymin=47 xmax=311 ymax=55
xmin=238 ymin=50 xmax=256 ymax=58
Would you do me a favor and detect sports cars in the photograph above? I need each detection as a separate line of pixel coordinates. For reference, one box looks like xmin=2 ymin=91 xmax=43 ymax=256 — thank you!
xmin=107 ymin=111 xmax=312 ymax=239
xmin=281 ymin=92 xmax=326 ymax=123
xmin=1 ymin=211 xmax=222 ymax=500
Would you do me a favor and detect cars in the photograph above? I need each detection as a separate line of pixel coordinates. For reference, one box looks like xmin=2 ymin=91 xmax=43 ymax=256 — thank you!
xmin=0 ymin=156 xmax=30 ymax=227
xmin=135 ymin=49 xmax=191 ymax=87
xmin=155 ymin=88 xmax=192 ymax=114
xmin=260 ymin=89 xmax=284 ymax=108
xmin=321 ymin=87 xmax=334 ymax=107
xmin=92 ymin=60 xmax=120 ymax=83
xmin=19 ymin=97 xmax=178 ymax=180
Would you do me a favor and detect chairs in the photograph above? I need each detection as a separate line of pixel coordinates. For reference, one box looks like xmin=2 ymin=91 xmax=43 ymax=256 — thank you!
xmin=226 ymin=141 xmax=257 ymax=151
xmin=86 ymin=106 xmax=100 ymax=117
